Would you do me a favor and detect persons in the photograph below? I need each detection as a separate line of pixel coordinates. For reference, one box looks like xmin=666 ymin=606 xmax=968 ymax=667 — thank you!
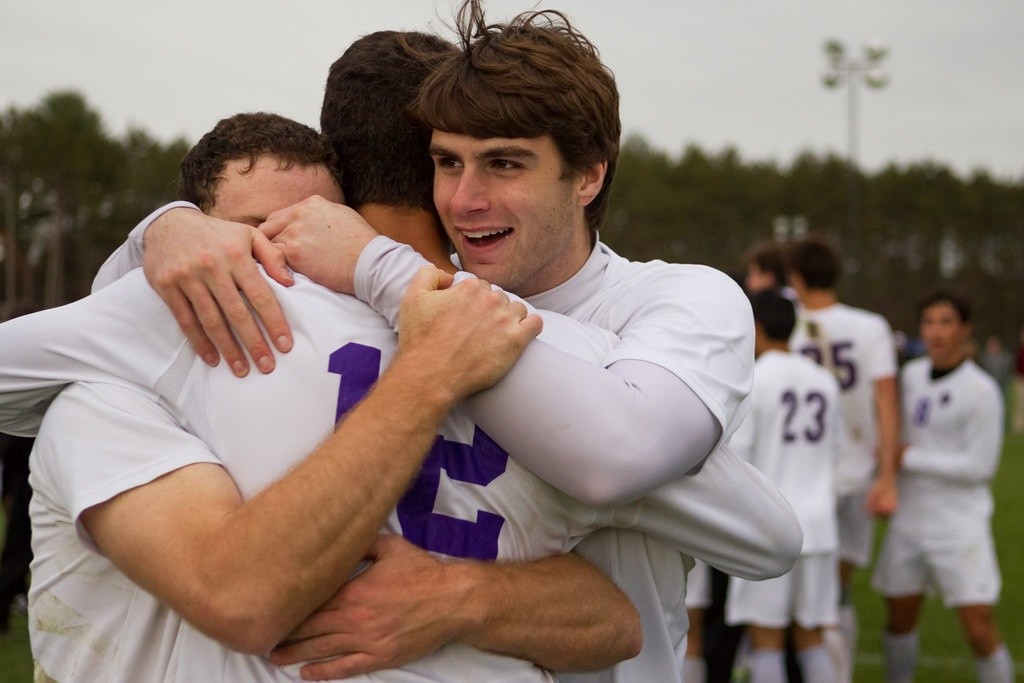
xmin=680 ymin=237 xmax=900 ymax=683
xmin=868 ymin=290 xmax=1017 ymax=683
xmin=1 ymin=0 xmax=803 ymax=683
